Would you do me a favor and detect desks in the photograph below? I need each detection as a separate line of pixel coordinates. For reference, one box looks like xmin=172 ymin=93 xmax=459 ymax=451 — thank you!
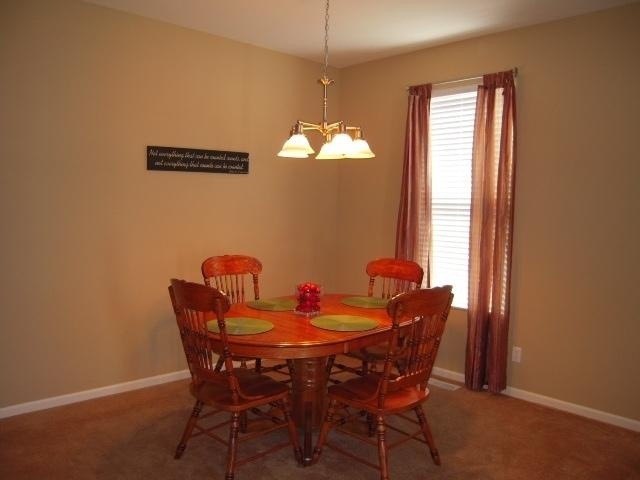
xmin=183 ymin=294 xmax=423 ymax=466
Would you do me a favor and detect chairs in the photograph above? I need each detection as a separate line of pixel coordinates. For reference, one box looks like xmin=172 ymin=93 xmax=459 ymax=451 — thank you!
xmin=201 ymin=254 xmax=294 ymax=423
xmin=312 ymin=284 xmax=454 ymax=480
xmin=325 ymin=258 xmax=425 ymax=425
xmin=167 ymin=277 xmax=303 ymax=480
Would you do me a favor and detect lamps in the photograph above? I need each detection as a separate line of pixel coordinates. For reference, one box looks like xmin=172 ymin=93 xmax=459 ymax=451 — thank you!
xmin=277 ymin=0 xmax=376 ymax=161
